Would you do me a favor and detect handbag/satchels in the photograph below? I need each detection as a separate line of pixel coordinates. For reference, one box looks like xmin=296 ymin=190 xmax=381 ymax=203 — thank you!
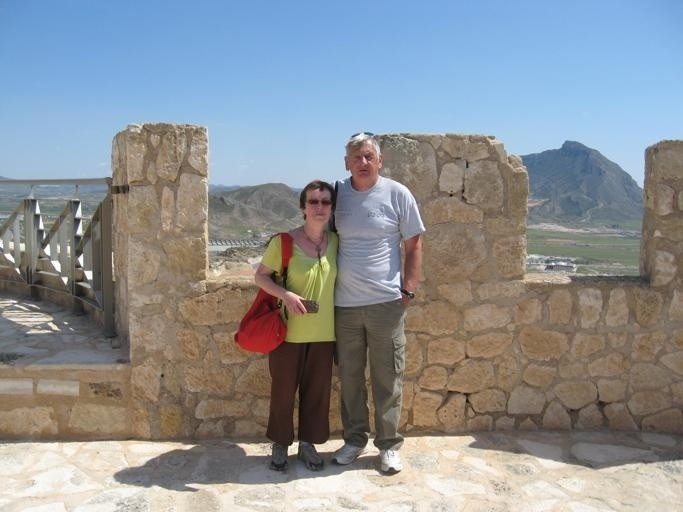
xmin=233 ymin=233 xmax=291 ymax=353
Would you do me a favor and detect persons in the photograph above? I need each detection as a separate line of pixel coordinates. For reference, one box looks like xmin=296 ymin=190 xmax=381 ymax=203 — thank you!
xmin=254 ymin=181 xmax=339 ymax=471
xmin=265 ymin=133 xmax=427 ymax=474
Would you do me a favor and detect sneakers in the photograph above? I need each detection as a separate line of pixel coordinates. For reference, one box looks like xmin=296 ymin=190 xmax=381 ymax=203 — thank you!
xmin=269 ymin=442 xmax=288 ymax=471
xmin=297 ymin=441 xmax=323 ymax=471
xmin=330 ymin=444 xmax=367 ymax=465
xmin=379 ymin=449 xmax=401 ymax=473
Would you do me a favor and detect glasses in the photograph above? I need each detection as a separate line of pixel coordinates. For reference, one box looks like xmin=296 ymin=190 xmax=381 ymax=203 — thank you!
xmin=308 ymin=199 xmax=331 ymax=204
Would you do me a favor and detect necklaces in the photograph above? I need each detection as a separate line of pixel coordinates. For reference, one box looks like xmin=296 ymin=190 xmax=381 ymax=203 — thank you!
xmin=301 ymin=226 xmax=327 ymax=268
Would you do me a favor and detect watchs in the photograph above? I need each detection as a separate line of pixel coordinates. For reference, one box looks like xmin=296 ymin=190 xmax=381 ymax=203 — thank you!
xmin=401 ymin=288 xmax=416 ymax=301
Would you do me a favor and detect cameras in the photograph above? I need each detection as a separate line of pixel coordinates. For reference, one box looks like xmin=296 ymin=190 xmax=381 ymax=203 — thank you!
xmin=299 ymin=299 xmax=320 ymax=314
